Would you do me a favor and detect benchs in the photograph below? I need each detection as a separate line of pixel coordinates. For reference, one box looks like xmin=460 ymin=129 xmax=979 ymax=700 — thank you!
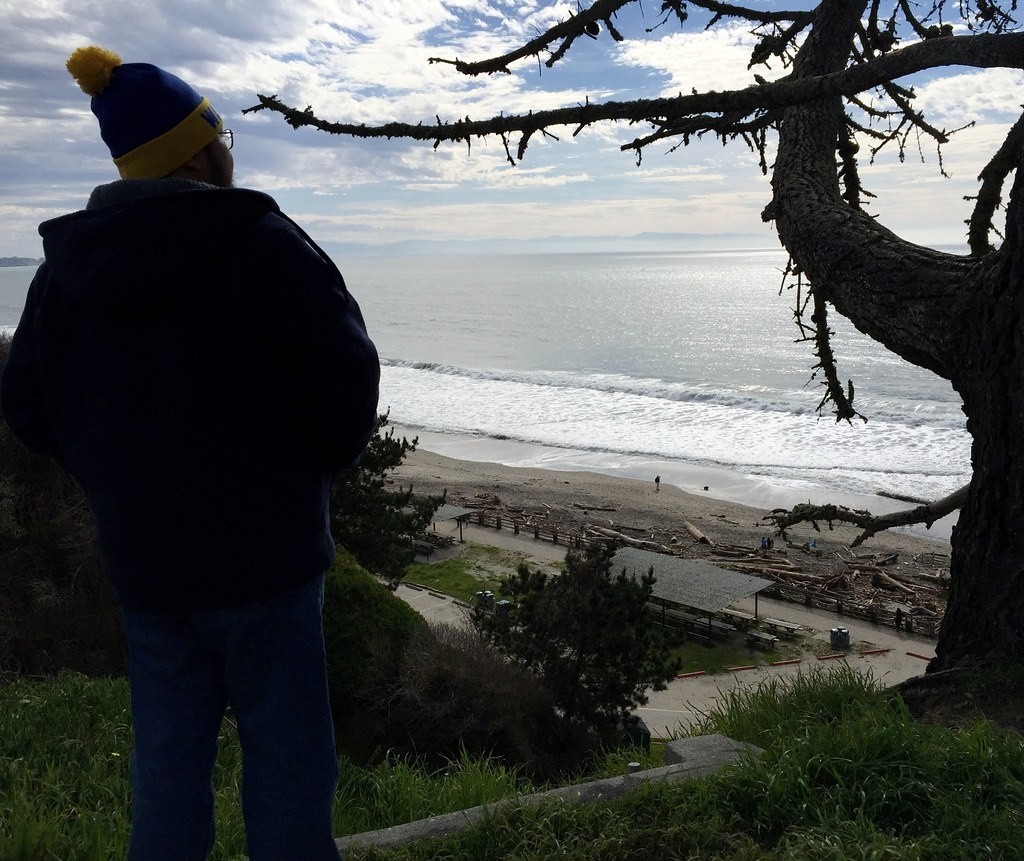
xmin=799 ymin=627 xmax=804 ymax=630
xmin=413 ymin=531 xmax=455 ymax=556
xmin=759 ymin=625 xmax=801 ymax=638
xmin=774 ymin=639 xmax=780 ymax=641
xmin=753 ymin=617 xmax=756 ymax=620
xmin=732 ymin=626 xmax=737 ymax=630
xmin=637 ymin=602 xmax=807 ymax=653
xmin=745 ymin=638 xmax=770 ymax=647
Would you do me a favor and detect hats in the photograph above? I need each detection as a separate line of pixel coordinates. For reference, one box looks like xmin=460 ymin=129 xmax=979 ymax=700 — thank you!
xmin=62 ymin=45 xmax=223 ymax=180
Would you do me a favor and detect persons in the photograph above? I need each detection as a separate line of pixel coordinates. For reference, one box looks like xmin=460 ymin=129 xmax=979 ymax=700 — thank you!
xmin=810 ymin=539 xmax=817 ymax=548
xmin=0 ymin=47 xmax=380 ymax=861
xmin=655 ymin=476 xmax=661 ymax=491
xmin=760 ymin=537 xmax=774 ymax=547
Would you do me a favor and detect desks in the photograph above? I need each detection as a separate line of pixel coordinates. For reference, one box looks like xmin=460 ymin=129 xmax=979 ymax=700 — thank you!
xmin=413 ymin=538 xmax=435 ymax=551
xmin=434 ymin=532 xmax=457 ymax=542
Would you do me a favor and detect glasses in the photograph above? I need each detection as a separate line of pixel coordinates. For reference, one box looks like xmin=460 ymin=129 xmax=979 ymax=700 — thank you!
xmin=218 ymin=128 xmax=234 ymax=151
